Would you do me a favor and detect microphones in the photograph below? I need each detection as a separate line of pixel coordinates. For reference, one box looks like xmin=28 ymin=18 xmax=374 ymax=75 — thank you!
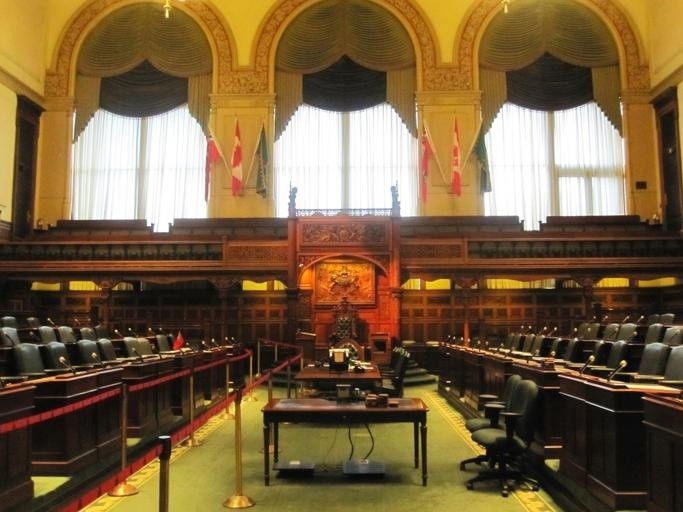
xmin=588 ymin=315 xmax=645 ymax=325
xmin=438 ymin=335 xmax=626 ymax=381
xmin=175 ymin=336 xmax=237 ymax=364
xmin=91 ymin=352 xmax=107 ymax=369
xmin=151 ymin=345 xmax=162 ymax=360
xmin=517 ymin=325 xmax=636 ymax=344
xmin=58 ymin=356 xmax=77 ymax=375
xmin=132 ymin=348 xmax=144 ymax=363
xmin=3 ymin=316 xmax=168 ymax=346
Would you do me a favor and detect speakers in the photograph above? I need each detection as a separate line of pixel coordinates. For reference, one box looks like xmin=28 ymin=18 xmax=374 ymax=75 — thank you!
xmin=636 ymin=181 xmax=647 ymax=189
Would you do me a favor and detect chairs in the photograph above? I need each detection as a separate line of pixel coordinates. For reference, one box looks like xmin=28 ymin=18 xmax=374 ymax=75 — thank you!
xmin=123 ymin=336 xmax=140 ymax=357
xmin=138 ymin=337 xmax=153 ymax=356
xmin=77 ymin=339 xmax=101 ymax=363
xmin=467 ymin=380 xmax=539 ymax=497
xmin=459 ymin=375 xmax=521 ymax=471
xmin=156 ymin=334 xmax=170 ymax=352
xmin=503 ymin=313 xmax=682 ymax=388
xmin=14 ymin=343 xmax=44 ymax=372
xmin=45 ymin=341 xmax=72 ymax=370
xmin=0 ymin=316 xmax=114 ymax=350
xmin=379 ymin=345 xmax=411 ymax=397
xmin=97 ymin=338 xmax=117 ymax=361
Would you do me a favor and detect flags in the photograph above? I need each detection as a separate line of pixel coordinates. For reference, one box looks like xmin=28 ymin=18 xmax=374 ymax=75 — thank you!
xmin=452 ymin=115 xmax=463 ymax=197
xmin=204 ymin=127 xmax=222 ymax=203
xmin=255 ymin=126 xmax=270 ymax=200
xmin=420 ymin=117 xmax=435 ymax=201
xmin=230 ymin=120 xmax=243 ymax=197
xmin=172 ymin=329 xmax=184 ymax=350
xmin=472 ymin=121 xmax=493 ymax=195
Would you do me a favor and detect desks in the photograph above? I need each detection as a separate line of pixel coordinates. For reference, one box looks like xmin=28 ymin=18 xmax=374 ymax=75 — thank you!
xmin=261 ymin=397 xmax=429 ymax=486
xmin=295 ymin=361 xmax=382 ymax=398
